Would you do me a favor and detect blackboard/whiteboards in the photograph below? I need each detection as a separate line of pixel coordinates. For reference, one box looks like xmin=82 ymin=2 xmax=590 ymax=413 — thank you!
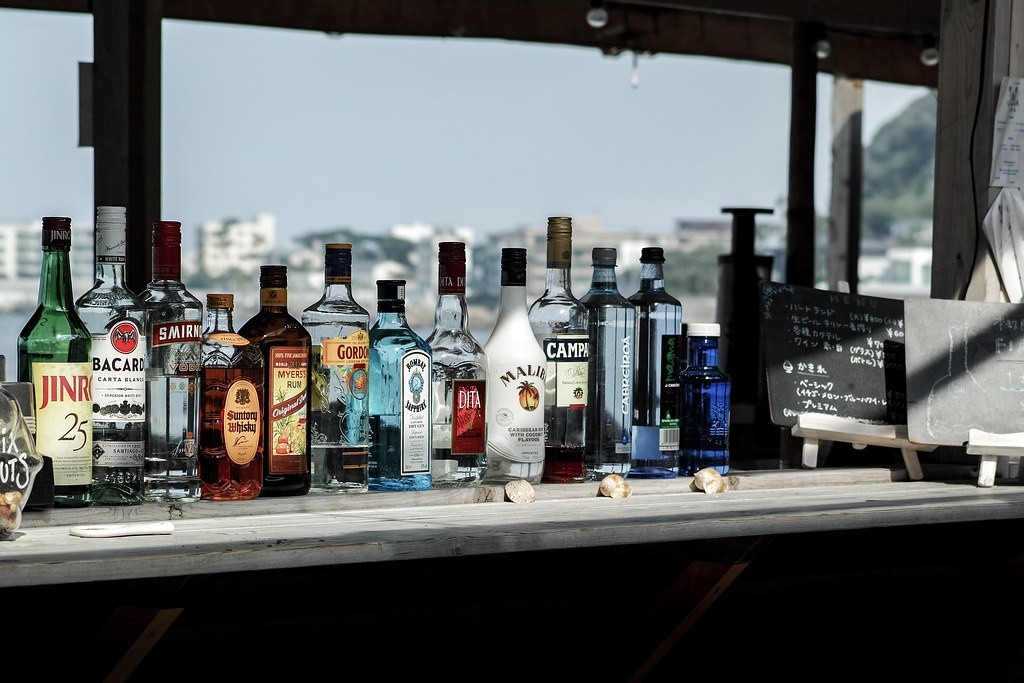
xmin=903 ymin=296 xmax=1024 ymax=457
xmin=756 ymin=280 xmax=939 ymax=452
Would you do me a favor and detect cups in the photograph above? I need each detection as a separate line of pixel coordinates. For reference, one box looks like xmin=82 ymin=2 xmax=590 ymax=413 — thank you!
xmin=0 ymin=381 xmax=37 ymax=435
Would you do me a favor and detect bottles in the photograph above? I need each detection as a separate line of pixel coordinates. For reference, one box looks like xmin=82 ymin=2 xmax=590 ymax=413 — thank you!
xmin=236 ymin=265 xmax=312 ymax=497
xmin=17 ymin=217 xmax=93 ymax=508
xmin=198 ymin=293 xmax=263 ymax=502
xmin=679 ymin=322 xmax=731 ymax=477
xmin=575 ymin=246 xmax=637 ymax=480
xmin=626 ymin=246 xmax=682 ymax=479
xmin=425 ymin=242 xmax=488 ymax=486
xmin=527 ymin=216 xmax=589 ymax=484
xmin=136 ymin=221 xmax=204 ymax=504
xmin=303 ymin=242 xmax=371 ymax=495
xmin=72 ymin=206 xmax=144 ymax=505
xmin=481 ymin=247 xmax=548 ymax=485
xmin=369 ymin=280 xmax=433 ymax=491
